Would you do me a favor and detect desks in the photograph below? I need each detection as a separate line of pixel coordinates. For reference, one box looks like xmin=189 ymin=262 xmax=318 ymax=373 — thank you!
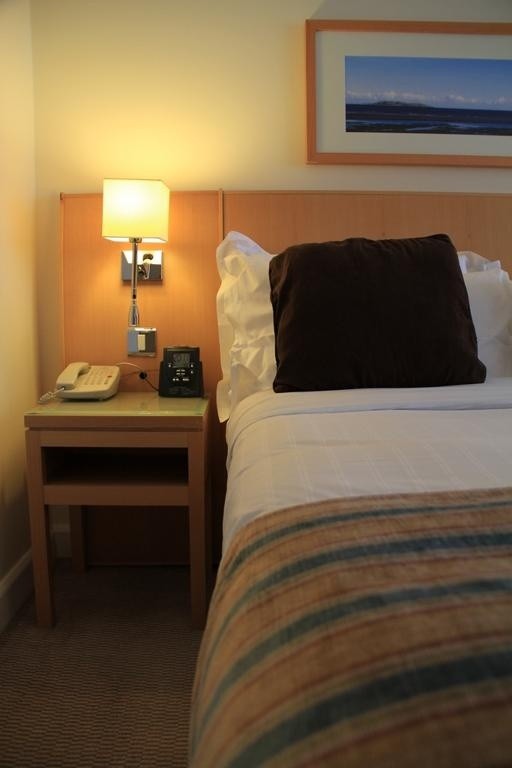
xmin=23 ymin=387 xmax=209 ymax=630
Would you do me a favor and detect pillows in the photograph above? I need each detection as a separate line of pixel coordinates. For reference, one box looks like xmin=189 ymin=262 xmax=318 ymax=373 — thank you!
xmin=262 ymin=226 xmax=487 ymax=393
xmin=210 ymin=224 xmax=508 ymax=422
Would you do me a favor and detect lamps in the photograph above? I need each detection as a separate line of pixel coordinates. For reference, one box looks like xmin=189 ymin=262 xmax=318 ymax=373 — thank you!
xmin=96 ymin=176 xmax=171 ymax=359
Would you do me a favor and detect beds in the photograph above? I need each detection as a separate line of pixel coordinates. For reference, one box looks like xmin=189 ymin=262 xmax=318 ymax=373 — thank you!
xmin=189 ymin=190 xmax=510 ymax=768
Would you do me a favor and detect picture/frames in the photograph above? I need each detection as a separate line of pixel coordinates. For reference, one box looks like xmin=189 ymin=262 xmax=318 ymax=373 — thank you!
xmin=297 ymin=14 xmax=511 ymax=168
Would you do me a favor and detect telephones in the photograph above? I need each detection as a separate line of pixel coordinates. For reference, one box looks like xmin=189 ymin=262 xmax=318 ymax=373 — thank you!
xmin=54 ymin=362 xmax=121 ymax=401
